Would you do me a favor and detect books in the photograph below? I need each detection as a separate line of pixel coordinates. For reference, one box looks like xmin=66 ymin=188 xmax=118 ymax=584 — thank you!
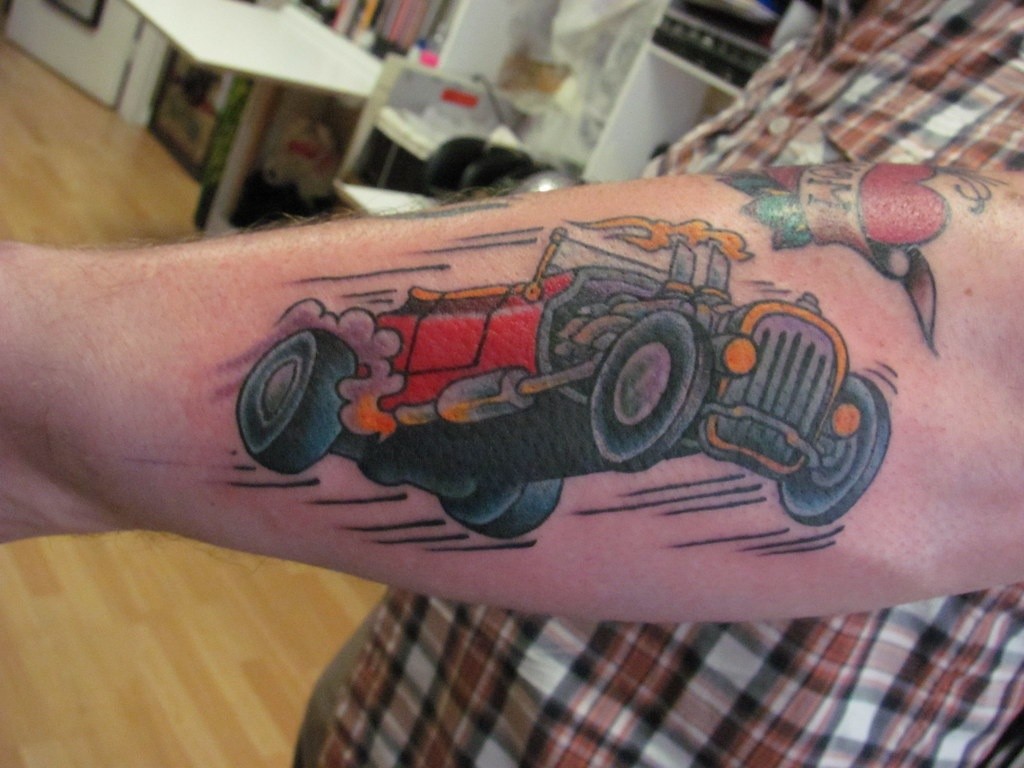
xmin=298 ymin=0 xmax=460 ymax=54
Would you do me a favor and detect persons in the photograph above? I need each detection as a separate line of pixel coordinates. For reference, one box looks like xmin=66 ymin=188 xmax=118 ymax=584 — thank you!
xmin=0 ymin=0 xmax=1024 ymax=768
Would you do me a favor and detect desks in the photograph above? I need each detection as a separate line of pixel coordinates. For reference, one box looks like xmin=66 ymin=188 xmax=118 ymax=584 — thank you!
xmin=127 ymin=0 xmax=386 ymax=232
